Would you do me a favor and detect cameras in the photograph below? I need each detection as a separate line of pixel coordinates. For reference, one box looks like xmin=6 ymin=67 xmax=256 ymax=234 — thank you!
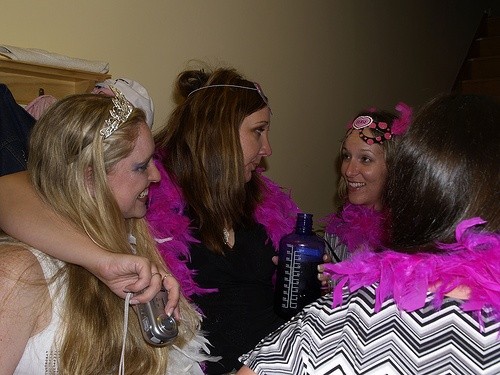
xmin=133 ymin=283 xmax=178 ymax=346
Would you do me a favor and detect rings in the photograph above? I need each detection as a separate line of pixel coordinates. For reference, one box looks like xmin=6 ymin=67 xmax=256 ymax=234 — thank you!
xmin=328 ymin=279 xmax=333 ymax=287
xmin=152 ymin=272 xmax=162 ymax=278
xmin=162 ymin=273 xmax=173 ymax=283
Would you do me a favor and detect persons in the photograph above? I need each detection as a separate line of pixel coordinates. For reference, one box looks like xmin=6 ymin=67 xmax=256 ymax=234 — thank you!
xmin=0 ymin=93 xmax=205 ymax=375
xmin=1 ymin=63 xmax=333 ymax=375
xmin=320 ymin=108 xmax=411 ymax=293
xmin=231 ymin=87 xmax=498 ymax=375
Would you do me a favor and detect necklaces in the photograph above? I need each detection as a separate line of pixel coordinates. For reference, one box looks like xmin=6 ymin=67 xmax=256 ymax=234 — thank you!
xmin=220 ymin=223 xmax=231 ymax=246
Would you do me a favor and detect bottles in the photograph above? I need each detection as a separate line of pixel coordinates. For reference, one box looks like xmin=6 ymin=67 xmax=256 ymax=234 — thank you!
xmin=276 ymin=212 xmax=325 ymax=320
